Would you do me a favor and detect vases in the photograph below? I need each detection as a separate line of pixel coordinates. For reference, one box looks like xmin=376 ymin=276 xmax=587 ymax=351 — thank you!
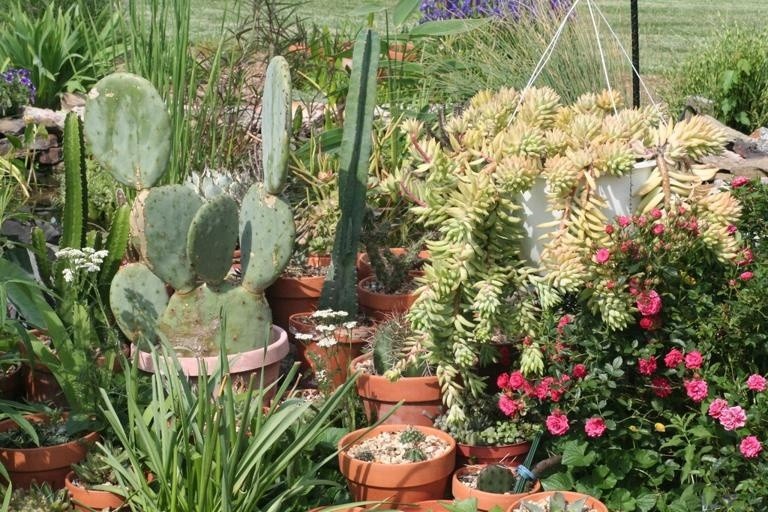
xmin=307 ymin=506 xmax=370 ymax=512
xmin=93 ymin=340 xmax=130 ymax=372
xmin=232 ymin=249 xmax=240 ymax=272
xmin=397 ymin=499 xmax=455 ymax=512
xmin=359 ymin=247 xmax=431 ymax=282
xmin=305 ymin=256 xmax=331 ymax=268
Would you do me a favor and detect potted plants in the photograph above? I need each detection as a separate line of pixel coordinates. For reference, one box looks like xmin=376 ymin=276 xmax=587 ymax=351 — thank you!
xmin=290 ymin=27 xmax=376 ymax=392
xmin=350 ymin=313 xmax=462 ymax=427
xmin=19 ymin=111 xmax=132 ymax=413
xmin=64 ymin=437 xmax=157 ymax=512
xmin=358 ymin=227 xmax=430 ymax=321
xmin=451 ymin=462 xmax=541 ymax=512
xmin=507 ymin=490 xmax=609 ymax=512
xmin=271 ymin=254 xmax=326 ymax=345
xmin=1 ymin=276 xmax=66 ymax=400
xmin=388 ymin=43 xmax=417 ymax=61
xmin=1 ymin=400 xmax=103 ymax=494
xmin=433 ymin=392 xmax=529 ymax=467
xmin=109 ymin=182 xmax=297 ymax=427
xmin=338 ymin=423 xmax=456 ymax=512
xmin=385 ymin=84 xmax=745 ymax=410
